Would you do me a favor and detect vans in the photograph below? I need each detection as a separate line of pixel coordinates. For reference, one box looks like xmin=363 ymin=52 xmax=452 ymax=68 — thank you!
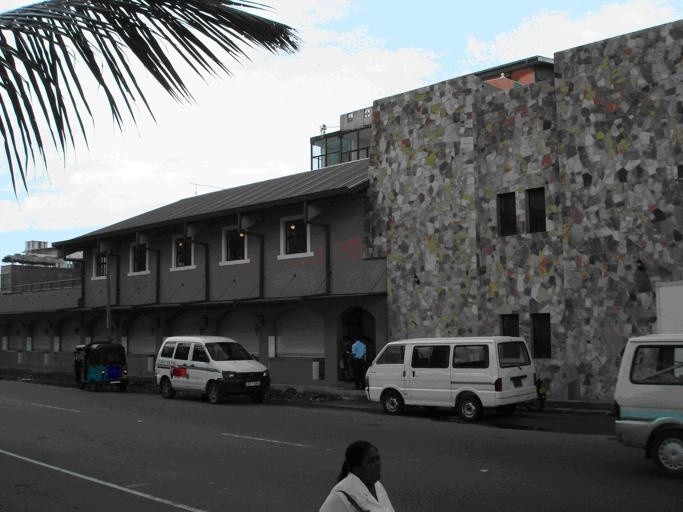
xmin=613 ymin=332 xmax=683 ymax=472
xmin=154 ymin=336 xmax=271 ymax=403
xmin=74 ymin=342 xmax=128 ymax=393
xmin=364 ymin=334 xmax=543 ymax=421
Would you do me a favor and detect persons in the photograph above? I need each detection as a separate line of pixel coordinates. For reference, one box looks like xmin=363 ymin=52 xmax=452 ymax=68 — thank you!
xmin=319 ymin=440 xmax=396 ymax=512
xmin=341 ymin=333 xmax=376 ymax=390
